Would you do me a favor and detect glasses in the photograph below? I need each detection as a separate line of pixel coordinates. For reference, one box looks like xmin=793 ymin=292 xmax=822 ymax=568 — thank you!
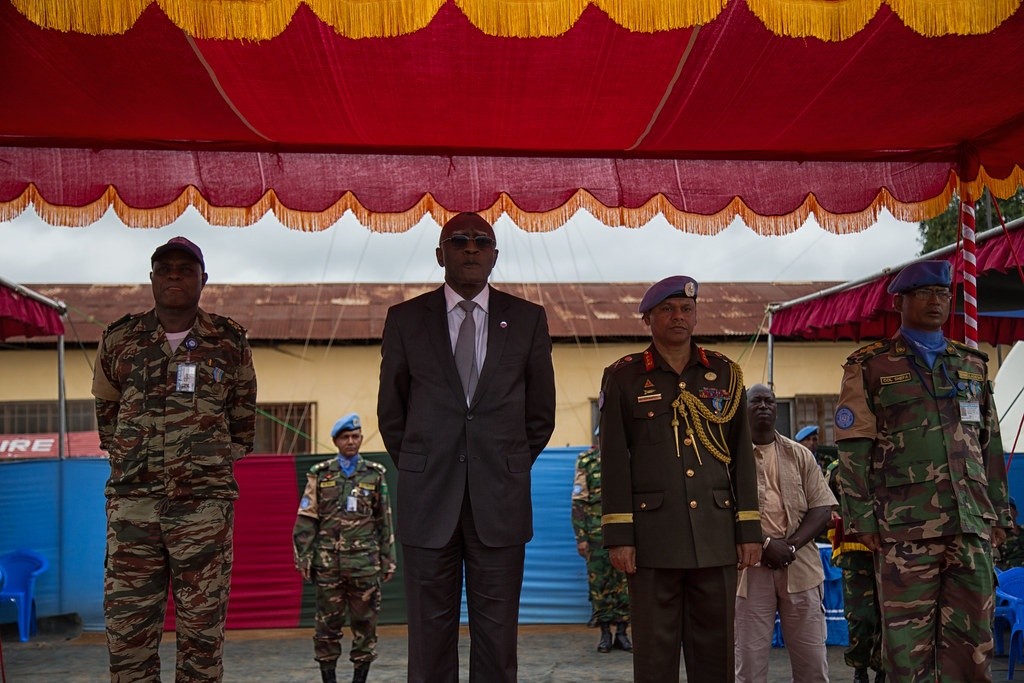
xmin=440 ymin=235 xmax=496 ymax=249
xmin=902 ymin=288 xmax=953 ymax=303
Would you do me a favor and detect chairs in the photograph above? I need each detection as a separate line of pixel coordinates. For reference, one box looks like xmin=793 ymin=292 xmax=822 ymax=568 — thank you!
xmin=0 ymin=549 xmax=48 ymax=643
xmin=991 ymin=564 xmax=1024 ymax=682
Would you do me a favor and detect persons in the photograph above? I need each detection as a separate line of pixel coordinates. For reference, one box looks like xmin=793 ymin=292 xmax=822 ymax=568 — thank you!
xmin=92 ymin=236 xmax=257 ymax=682
xmin=794 ymin=424 xmax=820 ymax=450
xmin=824 ymin=459 xmax=887 ymax=683
xmin=293 ymin=415 xmax=397 ymax=683
xmin=576 ymin=444 xmax=598 ymax=629
xmin=990 ymin=496 xmax=1024 ymax=573
xmin=834 ymin=259 xmax=1016 ymax=683
xmin=734 ymin=384 xmax=840 ymax=683
xmin=377 ymin=212 xmax=556 ymax=683
xmin=572 ymin=424 xmax=633 ymax=653
xmin=599 ymin=275 xmax=764 ymax=683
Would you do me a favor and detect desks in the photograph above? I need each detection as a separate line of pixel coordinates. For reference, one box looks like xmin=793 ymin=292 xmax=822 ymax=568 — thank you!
xmin=771 ymin=541 xmax=851 ymax=647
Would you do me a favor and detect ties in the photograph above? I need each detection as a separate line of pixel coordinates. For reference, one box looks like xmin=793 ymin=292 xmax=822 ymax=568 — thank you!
xmin=455 ymin=301 xmax=478 ymax=406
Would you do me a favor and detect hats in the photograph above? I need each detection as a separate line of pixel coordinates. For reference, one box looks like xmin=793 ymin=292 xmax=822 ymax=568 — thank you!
xmin=151 ymin=237 xmax=203 ymax=264
xmin=795 ymin=425 xmax=820 ymax=441
xmin=331 ymin=414 xmax=360 ymax=436
xmin=888 ymin=260 xmax=952 ymax=293
xmin=639 ymin=276 xmax=698 ymax=312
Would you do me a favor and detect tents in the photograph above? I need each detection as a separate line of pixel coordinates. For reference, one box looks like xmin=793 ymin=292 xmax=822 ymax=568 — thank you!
xmin=0 ymin=277 xmax=68 ymax=459
xmin=763 ymin=216 xmax=1024 ymax=394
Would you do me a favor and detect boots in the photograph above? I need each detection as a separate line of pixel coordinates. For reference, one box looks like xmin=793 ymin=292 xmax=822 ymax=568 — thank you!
xmin=613 ymin=621 xmax=634 ymax=653
xmin=874 ymin=671 xmax=886 ymax=683
xmin=853 ymin=667 xmax=869 ymax=683
xmin=597 ymin=621 xmax=612 ymax=653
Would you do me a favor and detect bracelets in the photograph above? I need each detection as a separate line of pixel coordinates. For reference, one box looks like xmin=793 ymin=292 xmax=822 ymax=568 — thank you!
xmin=785 ymin=541 xmax=796 ymax=554
xmin=763 ymin=536 xmax=770 ymax=549
